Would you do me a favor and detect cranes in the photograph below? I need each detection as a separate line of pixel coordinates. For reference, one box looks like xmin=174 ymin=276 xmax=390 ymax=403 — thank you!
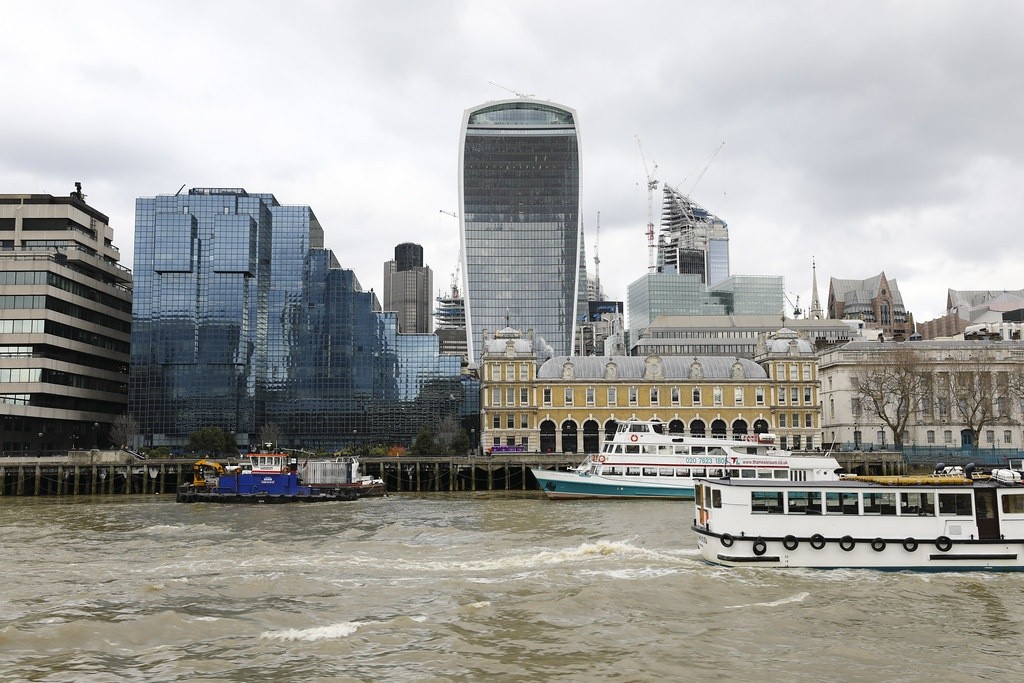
xmin=636 ymin=135 xmax=658 ymax=271
xmin=594 ymin=210 xmax=606 ymax=301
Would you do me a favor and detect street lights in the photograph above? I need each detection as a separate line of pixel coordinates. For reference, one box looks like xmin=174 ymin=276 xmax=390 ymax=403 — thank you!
xmin=352 ymin=428 xmax=359 ymax=459
xmin=757 ymin=422 xmax=762 ymax=435
xmin=854 ymin=422 xmax=861 ymax=452
xmin=468 ymin=427 xmax=476 ymax=458
xmin=90 ymin=421 xmax=99 ymax=452
xmin=565 ymin=423 xmax=572 ymax=455
xmin=228 ymin=431 xmax=236 ymax=457
xmin=37 ymin=433 xmax=43 ymax=458
xmin=879 ymin=424 xmax=887 ymax=452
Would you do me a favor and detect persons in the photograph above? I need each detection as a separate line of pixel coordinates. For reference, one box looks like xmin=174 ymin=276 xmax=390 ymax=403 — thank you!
xmin=773 ymin=446 xmax=776 ymax=450
xmin=870 ymin=445 xmax=873 ymax=450
xmin=912 ymin=444 xmax=916 ymax=453
xmin=881 ymin=443 xmax=886 ymax=450
xmin=965 ymin=462 xmax=976 ymax=480
xmin=787 ymin=447 xmax=793 ymax=451
xmin=814 ymin=447 xmax=820 ymax=452
xmin=805 ymin=448 xmax=809 ymax=452
xmin=838 ymin=447 xmax=841 ymax=451
xmin=535 ymin=449 xmax=538 ymax=454
xmin=854 ymin=444 xmax=860 ymax=450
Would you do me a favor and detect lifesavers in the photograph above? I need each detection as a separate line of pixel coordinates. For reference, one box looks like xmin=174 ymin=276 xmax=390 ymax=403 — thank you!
xmin=321 ymin=496 xmax=327 ymax=502
xmin=176 ymin=490 xmax=189 ymax=503
xmin=251 ymin=495 xmax=257 ymax=502
xmin=935 ymin=536 xmax=952 ymax=552
xmin=839 ymin=535 xmax=855 ymax=551
xmin=902 ymin=537 xmax=918 ymax=552
xmin=630 ymin=435 xmax=638 ymax=442
xmin=348 ymin=495 xmax=353 ymax=500
xmin=335 ymin=495 xmax=341 ymax=501
xmin=307 ymin=496 xmax=313 ymax=502
xmin=291 ymin=495 xmax=297 ymax=502
xmin=752 ymin=541 xmax=767 ymax=555
xmin=871 ymin=538 xmax=886 ymax=552
xmin=720 ymin=533 xmax=733 ymax=547
xmin=191 ymin=494 xmax=227 ymax=502
xmin=265 ymin=495 xmax=272 ymax=502
xmin=235 ymin=494 xmax=241 ymax=501
xmin=279 ymin=494 xmax=285 ymax=501
xmin=599 ymin=455 xmax=605 ymax=462
xmin=810 ymin=534 xmax=825 ymax=550
xmin=783 ymin=535 xmax=798 ymax=551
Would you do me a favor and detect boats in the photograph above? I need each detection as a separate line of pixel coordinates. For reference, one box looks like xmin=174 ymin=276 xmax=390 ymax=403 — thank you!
xmin=688 ymin=457 xmax=1024 ymax=575
xmin=527 ymin=417 xmax=843 ymax=501
xmin=177 ymin=442 xmax=388 ymax=504
